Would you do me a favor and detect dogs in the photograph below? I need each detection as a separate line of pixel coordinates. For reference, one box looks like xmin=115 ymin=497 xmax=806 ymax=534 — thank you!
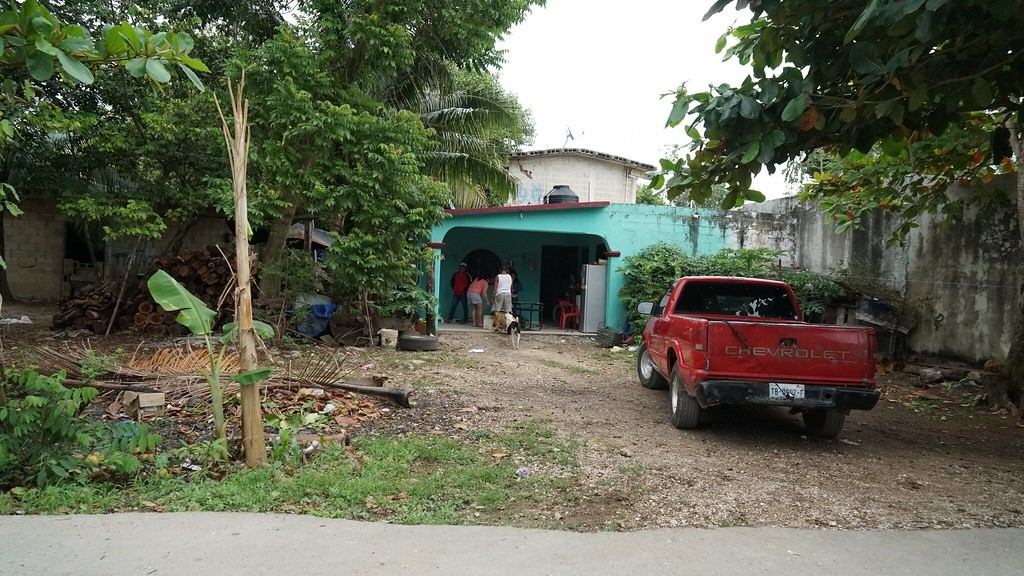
xmin=493 ymin=310 xmax=521 ymax=350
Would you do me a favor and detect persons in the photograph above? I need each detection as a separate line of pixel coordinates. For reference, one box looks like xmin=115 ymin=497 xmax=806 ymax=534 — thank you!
xmin=565 ymin=272 xmax=580 ymax=302
xmin=490 ymin=265 xmax=513 ymax=330
xmin=446 ymin=262 xmax=472 ymax=324
xmin=466 ymin=273 xmax=491 ymax=326
xmin=509 ymin=271 xmax=522 ymax=317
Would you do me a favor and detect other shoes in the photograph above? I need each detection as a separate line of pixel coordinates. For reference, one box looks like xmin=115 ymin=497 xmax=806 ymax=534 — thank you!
xmin=479 ymin=325 xmax=483 ymax=327
xmin=444 ymin=319 xmax=452 ymax=323
xmin=495 ymin=328 xmax=499 ymax=331
xmin=460 ymin=321 xmax=467 ymax=324
xmin=472 ymin=324 xmax=477 ymax=327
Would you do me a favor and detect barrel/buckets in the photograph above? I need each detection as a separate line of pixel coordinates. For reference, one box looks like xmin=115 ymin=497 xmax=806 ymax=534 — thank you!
xmin=483 ymin=315 xmax=494 ymax=330
xmin=381 ymin=329 xmax=398 ymax=351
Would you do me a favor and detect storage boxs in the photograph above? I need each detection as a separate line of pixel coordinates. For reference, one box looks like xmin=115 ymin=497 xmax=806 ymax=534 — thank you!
xmin=482 ymin=314 xmax=496 ymax=330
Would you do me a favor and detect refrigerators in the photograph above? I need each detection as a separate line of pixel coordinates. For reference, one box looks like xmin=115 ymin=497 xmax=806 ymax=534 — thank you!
xmin=579 ymin=264 xmax=606 ymax=332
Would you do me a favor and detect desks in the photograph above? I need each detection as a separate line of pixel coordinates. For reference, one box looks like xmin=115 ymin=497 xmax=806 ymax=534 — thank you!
xmin=507 ymin=301 xmax=545 ymax=331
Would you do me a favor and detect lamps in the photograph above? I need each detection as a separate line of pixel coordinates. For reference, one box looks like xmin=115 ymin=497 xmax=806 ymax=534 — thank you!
xmin=517 ymin=210 xmax=526 ymax=222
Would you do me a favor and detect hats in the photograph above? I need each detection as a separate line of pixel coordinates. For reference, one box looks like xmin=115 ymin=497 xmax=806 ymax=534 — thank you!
xmin=459 ymin=263 xmax=467 ymax=267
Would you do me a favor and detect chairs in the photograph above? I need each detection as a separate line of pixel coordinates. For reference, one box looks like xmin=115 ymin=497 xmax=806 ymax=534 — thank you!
xmin=558 ymin=297 xmax=581 ymax=331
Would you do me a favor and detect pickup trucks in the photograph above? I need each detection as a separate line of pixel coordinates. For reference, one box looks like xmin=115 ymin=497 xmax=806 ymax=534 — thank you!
xmin=637 ymin=275 xmax=881 ymax=444
